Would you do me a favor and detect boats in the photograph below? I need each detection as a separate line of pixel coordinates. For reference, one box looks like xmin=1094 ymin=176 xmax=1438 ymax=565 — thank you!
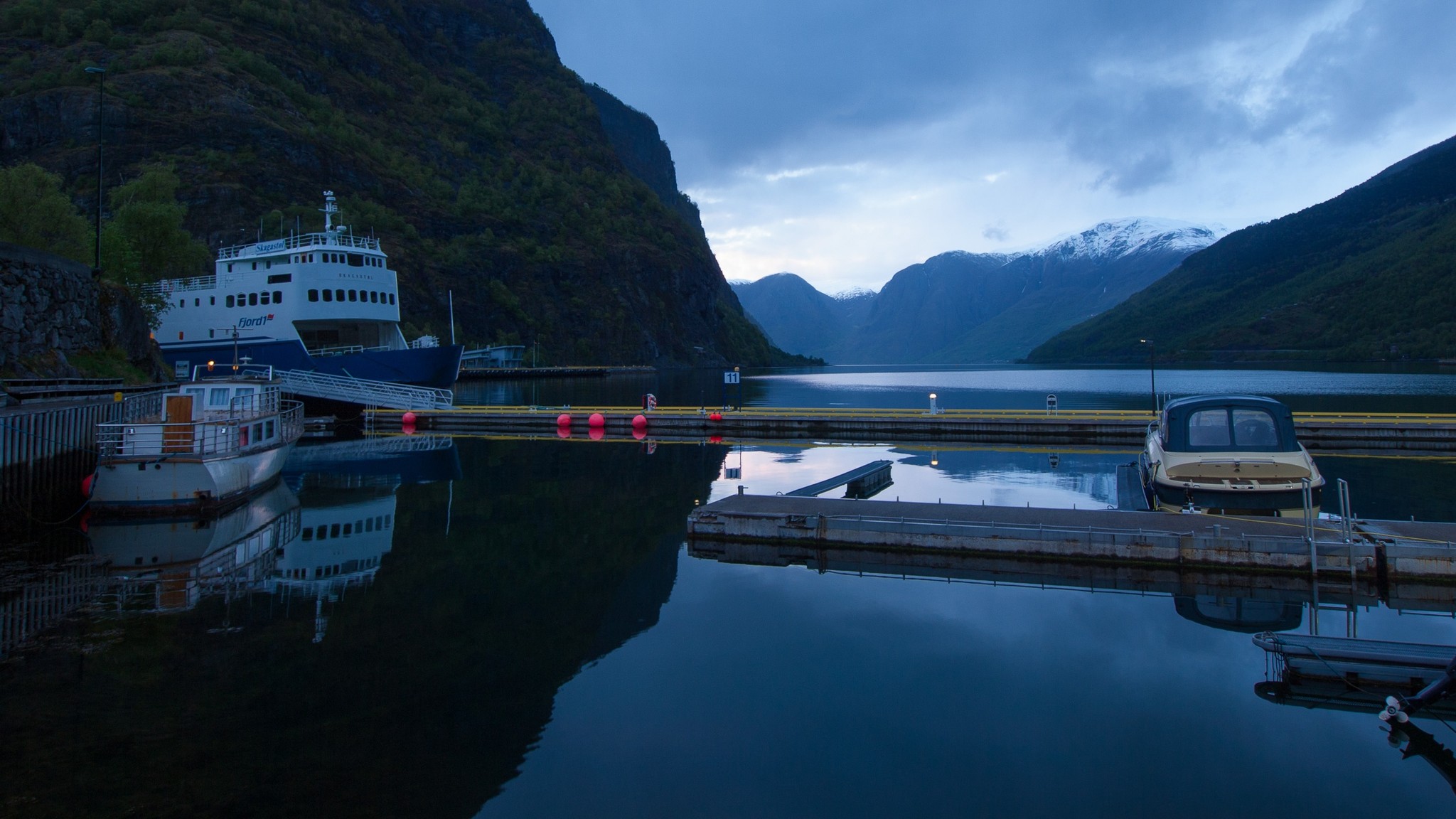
xmin=129 ymin=191 xmax=470 ymax=405
xmin=461 ymin=346 xmax=493 ymax=369
xmin=77 ymin=475 xmax=302 ymax=618
xmin=491 ymin=346 xmax=526 ymax=369
xmin=1136 ymin=393 xmax=1330 ymax=522
xmin=260 ymin=434 xmax=465 ymax=648
xmin=89 ymin=378 xmax=316 ymax=519
xmin=1253 ymin=630 xmax=1456 ymax=693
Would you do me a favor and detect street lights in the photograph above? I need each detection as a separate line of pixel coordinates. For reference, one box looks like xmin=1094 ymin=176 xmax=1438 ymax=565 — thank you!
xmin=84 ymin=65 xmax=105 ymax=269
